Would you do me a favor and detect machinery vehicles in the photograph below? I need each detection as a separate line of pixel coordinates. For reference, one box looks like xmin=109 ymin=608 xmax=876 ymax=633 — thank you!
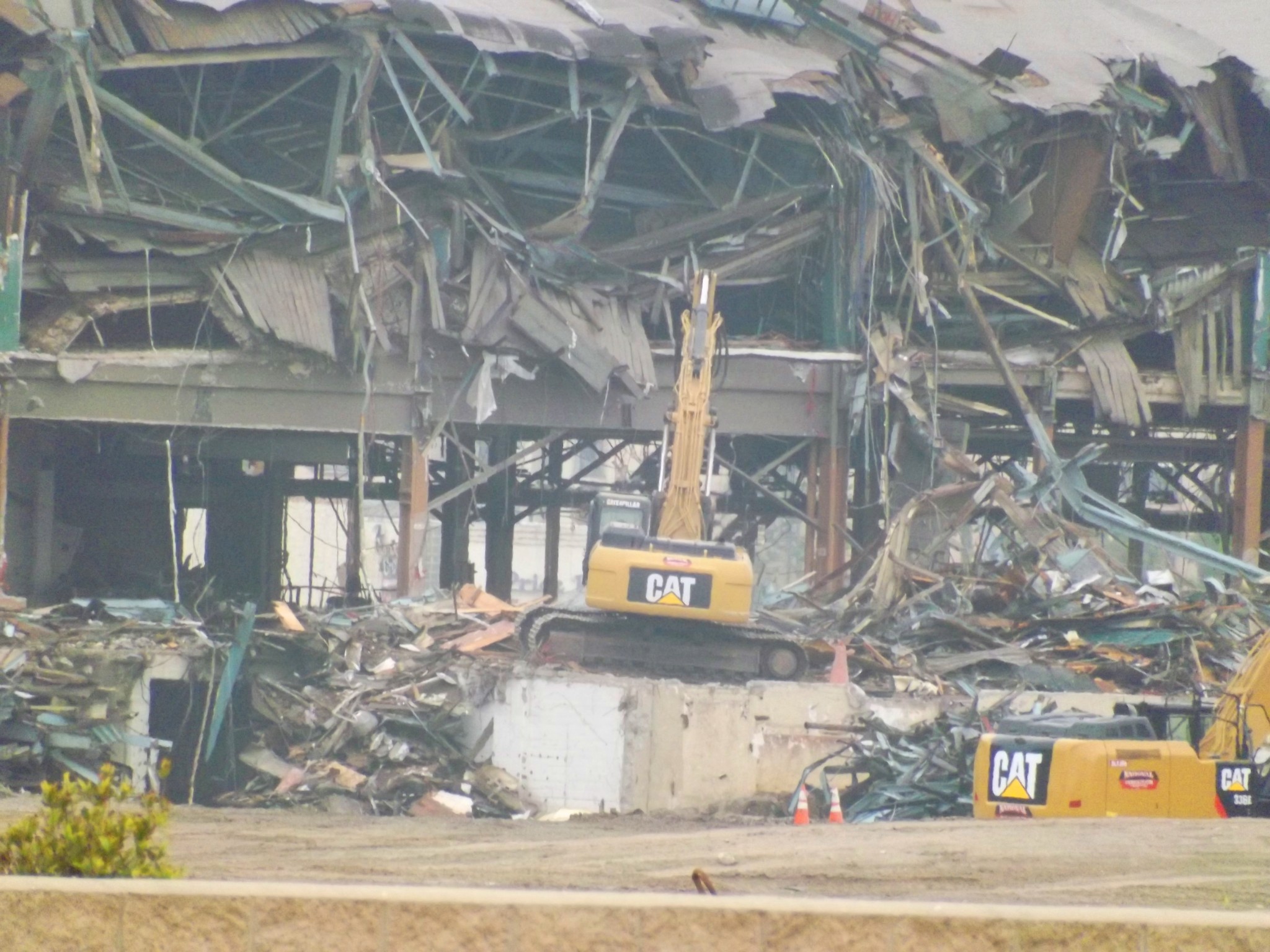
xmin=974 ymin=630 xmax=1270 ymax=820
xmin=514 ymin=269 xmax=811 ymax=682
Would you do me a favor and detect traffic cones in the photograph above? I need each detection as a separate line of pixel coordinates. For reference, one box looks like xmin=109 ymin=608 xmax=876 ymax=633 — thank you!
xmin=828 ymin=787 xmax=844 ymax=823
xmin=793 ymin=783 xmax=809 ymax=826
